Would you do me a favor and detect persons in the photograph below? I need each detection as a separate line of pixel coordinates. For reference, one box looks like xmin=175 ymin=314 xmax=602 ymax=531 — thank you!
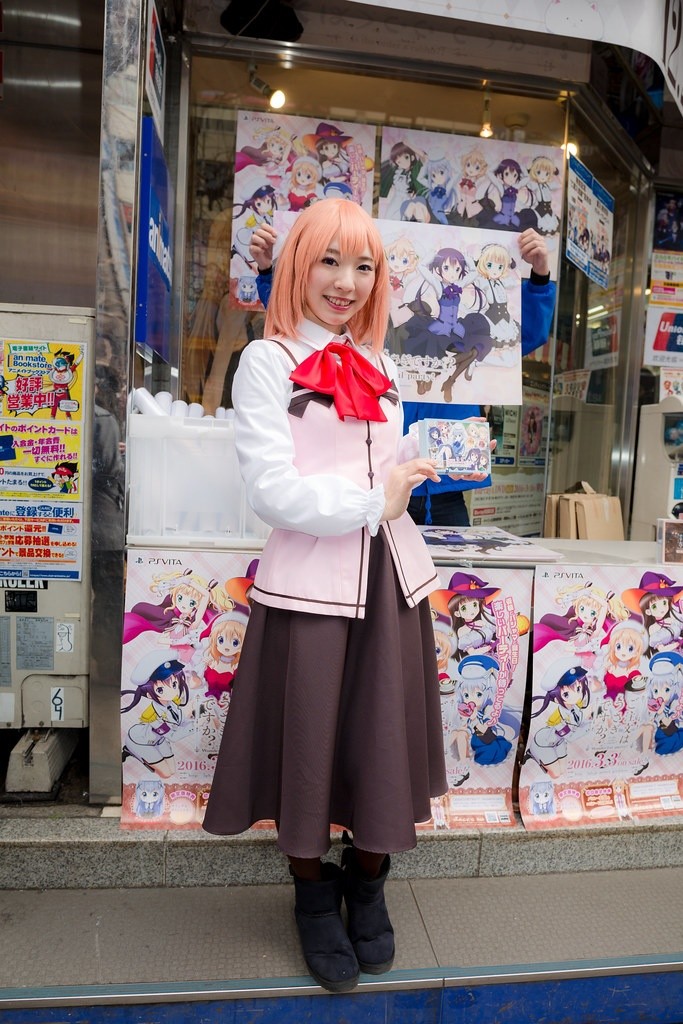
xmin=246 ymin=220 xmax=556 ymax=526
xmin=92 ymin=365 xmax=126 ymax=687
xmin=230 ymin=198 xmax=502 ymax=989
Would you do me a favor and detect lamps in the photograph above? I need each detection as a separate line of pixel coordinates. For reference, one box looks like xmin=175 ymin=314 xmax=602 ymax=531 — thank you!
xmin=248 ymin=61 xmax=286 ymax=107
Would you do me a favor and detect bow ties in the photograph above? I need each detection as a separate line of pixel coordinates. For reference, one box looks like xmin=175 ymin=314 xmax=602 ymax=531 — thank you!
xmin=290 ymin=341 xmax=392 ymax=423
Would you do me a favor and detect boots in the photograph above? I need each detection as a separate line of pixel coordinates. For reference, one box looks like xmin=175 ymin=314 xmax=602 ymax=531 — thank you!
xmin=289 ymin=847 xmax=395 ymax=991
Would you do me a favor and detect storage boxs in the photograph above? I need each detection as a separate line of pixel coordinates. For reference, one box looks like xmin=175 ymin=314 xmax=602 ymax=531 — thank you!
xmin=418 ymin=418 xmax=492 ymax=474
xmin=656 ymin=518 xmax=683 ymax=564
